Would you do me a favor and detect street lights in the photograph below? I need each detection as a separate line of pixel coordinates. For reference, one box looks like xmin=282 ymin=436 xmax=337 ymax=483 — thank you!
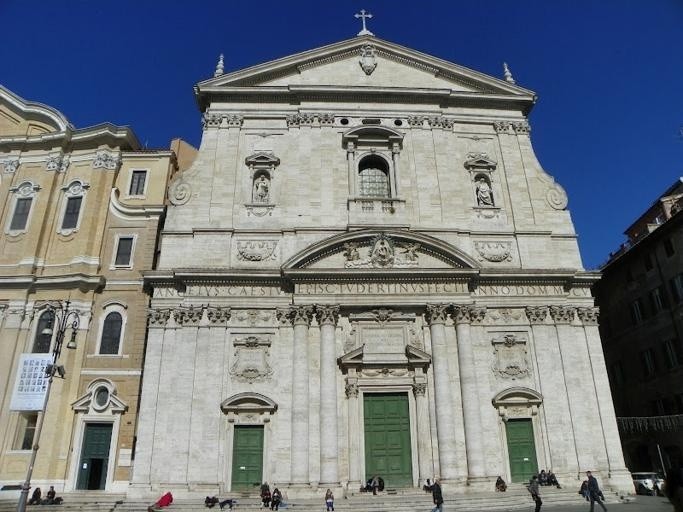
xmin=17 ymin=288 xmax=83 ymax=510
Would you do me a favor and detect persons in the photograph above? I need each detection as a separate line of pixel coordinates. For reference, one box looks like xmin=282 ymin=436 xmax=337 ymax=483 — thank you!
xmin=260 ymin=481 xmax=269 ymax=495
xmin=580 ymin=480 xmax=590 ymax=501
xmin=475 ymin=178 xmax=493 ymax=208
xmin=325 ymin=488 xmax=334 ymax=511
xmin=43 ymin=485 xmax=57 ymax=505
xmin=399 ymin=241 xmax=418 ymax=262
xmin=548 ymin=469 xmax=562 ymax=490
xmin=585 ymin=470 xmax=608 ymax=512
xmin=270 ymin=488 xmax=282 ymax=511
xmin=538 ymin=470 xmax=552 ymax=486
xmin=29 ymin=488 xmax=42 ymax=505
xmin=422 ymin=478 xmax=434 ymax=493
xmin=496 ymin=476 xmax=507 ymax=492
xmin=255 ymin=175 xmax=268 ymax=198
xmin=430 ymin=474 xmax=444 ymax=512
xmin=527 ymin=475 xmax=544 ymax=512
xmin=341 ymin=241 xmax=360 ymax=263
xmin=363 ymin=475 xmax=377 ymax=495
xmin=649 ymin=473 xmax=661 ymax=496
xmin=376 ymin=476 xmax=384 ymax=491
xmin=260 ymin=488 xmax=271 ymax=506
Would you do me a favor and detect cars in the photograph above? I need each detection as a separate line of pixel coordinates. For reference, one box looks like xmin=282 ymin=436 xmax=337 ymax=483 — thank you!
xmin=632 ymin=471 xmax=665 ymax=494
